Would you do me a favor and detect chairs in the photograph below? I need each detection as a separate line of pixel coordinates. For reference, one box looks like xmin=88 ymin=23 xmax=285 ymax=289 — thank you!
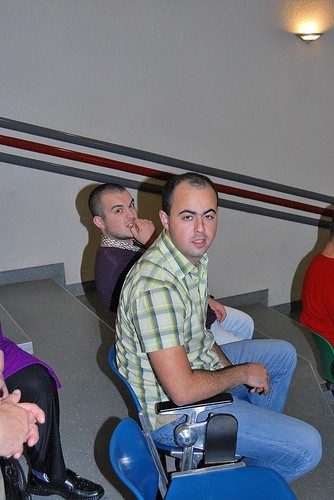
xmin=105 ymin=329 xmax=334 ymax=500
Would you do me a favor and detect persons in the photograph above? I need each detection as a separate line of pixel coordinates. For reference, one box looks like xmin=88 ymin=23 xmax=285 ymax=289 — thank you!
xmin=299 ymin=217 xmax=334 ymax=348
xmin=88 ymin=182 xmax=254 ymax=346
xmin=116 ymin=172 xmax=322 ymax=481
xmin=0 ymin=323 xmax=105 ymax=500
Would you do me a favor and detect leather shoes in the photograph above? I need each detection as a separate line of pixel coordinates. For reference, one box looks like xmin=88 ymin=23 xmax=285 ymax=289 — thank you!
xmin=28 ymin=467 xmax=105 ymax=500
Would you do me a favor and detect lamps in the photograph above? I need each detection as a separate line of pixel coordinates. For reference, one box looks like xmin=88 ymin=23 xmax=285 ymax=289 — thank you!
xmin=295 ymin=32 xmax=324 ymax=44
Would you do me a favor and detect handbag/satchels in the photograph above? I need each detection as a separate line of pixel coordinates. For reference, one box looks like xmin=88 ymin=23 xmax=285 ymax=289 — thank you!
xmin=0 ymin=457 xmax=33 ymax=500
